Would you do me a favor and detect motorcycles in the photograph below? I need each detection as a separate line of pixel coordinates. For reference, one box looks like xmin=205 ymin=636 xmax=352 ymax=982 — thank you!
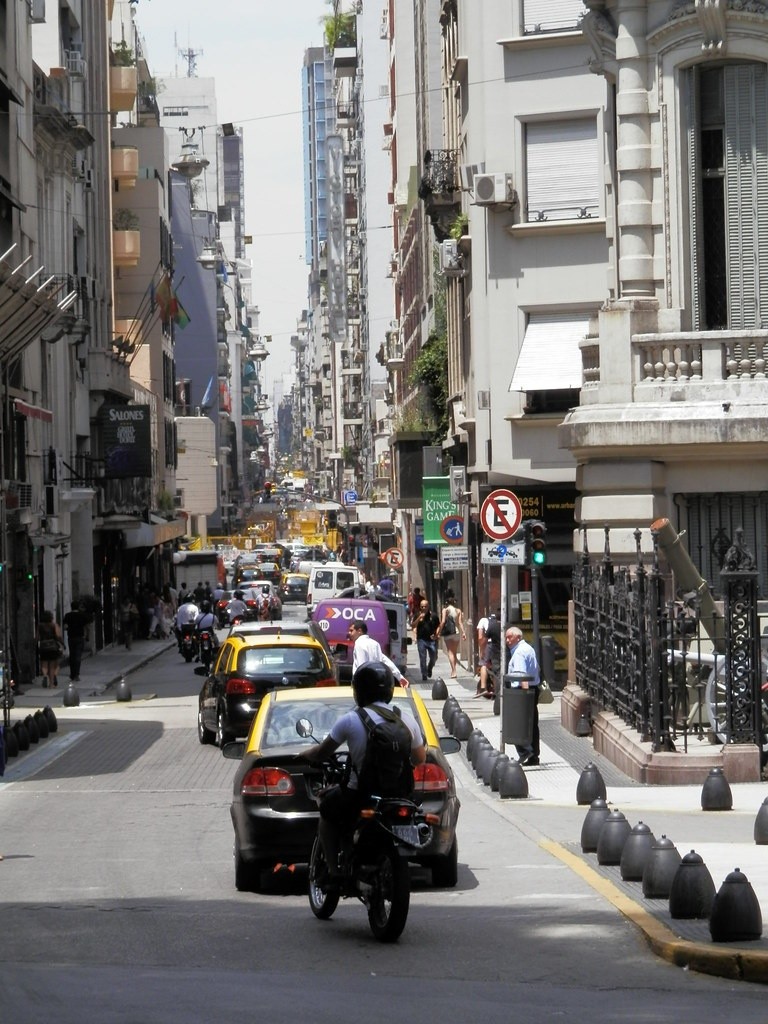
xmin=295 ymin=707 xmax=441 ymax=946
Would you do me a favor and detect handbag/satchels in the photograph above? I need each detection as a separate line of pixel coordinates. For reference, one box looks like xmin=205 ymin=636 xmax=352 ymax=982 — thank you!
xmin=536 ymin=680 xmax=554 ymax=704
xmin=440 ymin=615 xmax=456 ymax=636
xmin=130 ymin=603 xmax=140 ymax=621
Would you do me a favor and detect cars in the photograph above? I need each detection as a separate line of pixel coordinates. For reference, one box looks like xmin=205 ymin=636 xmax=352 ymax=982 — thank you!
xmin=196 ymin=620 xmax=337 ymax=750
xmin=215 ymin=544 xmax=328 ymax=629
xmin=230 ymin=684 xmax=463 ymax=895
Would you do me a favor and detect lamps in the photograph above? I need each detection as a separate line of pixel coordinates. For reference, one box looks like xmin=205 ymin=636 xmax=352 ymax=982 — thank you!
xmin=248 ymin=335 xmax=270 ymax=362
xmin=197 ymin=236 xmax=224 ymax=271
xmin=108 ymin=335 xmax=137 ymax=362
xmin=263 ymin=426 xmax=275 ymax=439
xmin=172 ymin=128 xmax=210 ymax=178
xmin=253 ymin=394 xmax=271 ymax=415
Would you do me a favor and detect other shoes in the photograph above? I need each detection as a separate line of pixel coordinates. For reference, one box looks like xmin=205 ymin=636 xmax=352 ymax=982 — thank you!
xmin=518 ymin=750 xmax=534 ymax=764
xmin=313 ymin=873 xmax=344 ymax=887
xmin=451 ymin=673 xmax=457 ymax=677
xmin=42 ymin=676 xmax=48 ymax=688
xmin=473 ymin=688 xmax=487 ymax=698
xmin=54 ymin=675 xmax=57 ymax=686
xmin=428 ymin=669 xmax=432 ymax=678
xmin=423 ymin=676 xmax=427 ymax=680
xmin=69 ymin=674 xmax=80 ymax=681
xmin=522 ymin=758 xmax=540 ymax=765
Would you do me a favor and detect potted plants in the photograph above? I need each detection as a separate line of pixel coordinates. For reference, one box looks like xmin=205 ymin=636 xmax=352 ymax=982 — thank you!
xmin=321 ymin=13 xmax=356 ymax=71
xmin=110 ymin=41 xmax=137 ymax=112
xmin=112 ymin=207 xmax=142 ymax=267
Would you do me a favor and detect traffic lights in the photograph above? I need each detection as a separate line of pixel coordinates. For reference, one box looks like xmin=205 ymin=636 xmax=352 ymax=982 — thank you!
xmin=529 ymin=522 xmax=546 ymax=566
xmin=326 ymin=510 xmax=337 ymax=530
xmin=264 ymin=482 xmax=272 ymax=500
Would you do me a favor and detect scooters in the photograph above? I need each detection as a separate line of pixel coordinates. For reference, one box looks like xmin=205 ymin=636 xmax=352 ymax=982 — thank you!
xmin=176 ymin=620 xmax=221 ymax=671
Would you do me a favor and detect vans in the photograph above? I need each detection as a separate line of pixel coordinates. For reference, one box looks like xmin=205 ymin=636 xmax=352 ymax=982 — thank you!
xmin=306 ymin=562 xmax=412 ymax=685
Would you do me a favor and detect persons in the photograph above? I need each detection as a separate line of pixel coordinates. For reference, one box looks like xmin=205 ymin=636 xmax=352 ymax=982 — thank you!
xmin=63 ymin=602 xmax=90 ymax=680
xmin=407 ymin=584 xmax=426 ymax=643
xmin=349 ymin=620 xmax=408 ymax=689
xmin=324 ymin=543 xmax=356 ymax=566
xmin=360 ymin=570 xmax=394 ymax=600
xmin=412 ymin=598 xmax=467 ymax=680
xmin=147 ymin=581 xmax=224 ymax=659
xmin=225 ymin=591 xmax=248 ymax=626
xmin=283 ymin=545 xmax=292 ymax=569
xmin=474 ymin=612 xmax=512 ymax=698
xmin=256 ymin=586 xmax=273 ymax=610
xmin=35 ymin=610 xmax=60 ymax=688
xmin=295 ymin=660 xmax=426 ymax=892
xmin=119 ymin=596 xmax=139 ymax=651
xmin=506 ymin=626 xmax=541 ymax=766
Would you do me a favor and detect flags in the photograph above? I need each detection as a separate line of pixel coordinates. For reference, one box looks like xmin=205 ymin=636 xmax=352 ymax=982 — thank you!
xmin=150 ymin=275 xmax=190 ymax=329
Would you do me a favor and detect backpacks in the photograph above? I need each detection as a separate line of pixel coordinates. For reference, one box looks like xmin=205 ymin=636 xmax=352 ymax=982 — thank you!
xmin=353 ymin=706 xmax=415 ymax=799
xmin=487 ymin=616 xmax=500 ymax=642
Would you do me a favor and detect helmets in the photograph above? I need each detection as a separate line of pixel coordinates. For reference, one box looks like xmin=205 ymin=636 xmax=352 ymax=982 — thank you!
xmin=200 ymin=600 xmax=211 ymax=612
xmin=262 ymin=586 xmax=269 ymax=592
xmin=217 ymin=583 xmax=223 ymax=589
xmin=351 ymin=660 xmax=394 ymax=707
xmin=234 ymin=591 xmax=246 ymax=600
xmin=185 ymin=593 xmax=196 ymax=602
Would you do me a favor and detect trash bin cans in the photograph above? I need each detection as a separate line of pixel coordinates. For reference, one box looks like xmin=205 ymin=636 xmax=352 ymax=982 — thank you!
xmin=501 ymin=673 xmax=537 ymax=745
xmin=82 ymin=610 xmax=96 ymax=654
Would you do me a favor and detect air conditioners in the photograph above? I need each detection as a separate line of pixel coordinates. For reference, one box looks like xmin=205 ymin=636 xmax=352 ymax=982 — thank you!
xmin=69 ymin=50 xmax=81 ymax=75
xmin=33 ymin=0 xmax=45 ymax=23
xmin=458 ymin=164 xmax=479 ymax=191
xmin=442 ymin=240 xmax=459 ymax=271
xmin=472 ymin=173 xmax=514 ymax=204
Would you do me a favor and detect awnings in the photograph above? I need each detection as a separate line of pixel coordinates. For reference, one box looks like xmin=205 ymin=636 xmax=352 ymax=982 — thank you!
xmin=508 ymin=315 xmax=595 ymax=391
